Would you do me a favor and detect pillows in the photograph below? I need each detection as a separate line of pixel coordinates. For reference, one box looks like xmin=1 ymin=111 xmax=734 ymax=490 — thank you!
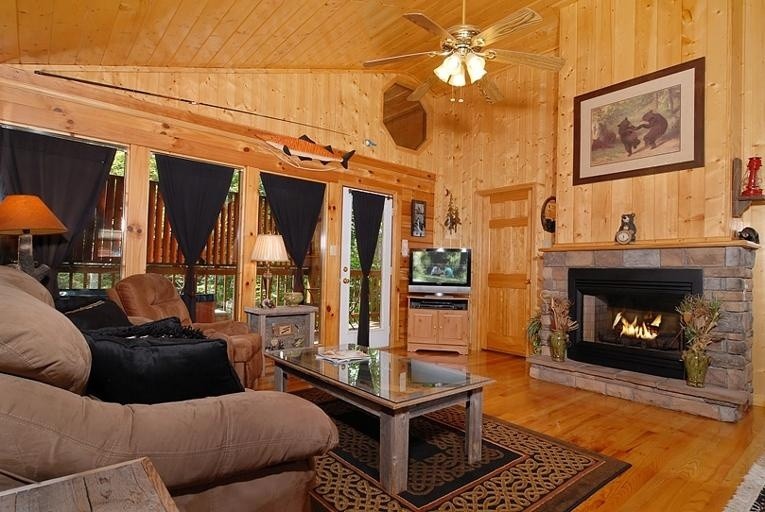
xmin=85 ymin=316 xmax=203 ymax=393
xmin=95 ymin=337 xmax=245 ymax=403
xmin=61 ymin=298 xmax=132 ymax=334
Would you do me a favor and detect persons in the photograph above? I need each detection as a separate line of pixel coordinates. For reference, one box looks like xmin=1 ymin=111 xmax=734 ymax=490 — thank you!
xmin=444 ymin=265 xmax=454 ymax=278
xmin=431 ymin=264 xmax=442 ymax=278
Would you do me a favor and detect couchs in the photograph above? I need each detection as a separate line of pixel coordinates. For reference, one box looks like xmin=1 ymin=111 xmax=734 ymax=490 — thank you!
xmin=0 ymin=265 xmax=340 ymax=512
xmin=107 ymin=273 xmax=262 ymax=389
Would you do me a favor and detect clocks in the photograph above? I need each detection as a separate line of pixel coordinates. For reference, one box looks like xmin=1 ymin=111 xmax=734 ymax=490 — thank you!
xmin=615 ymin=213 xmax=637 ymax=244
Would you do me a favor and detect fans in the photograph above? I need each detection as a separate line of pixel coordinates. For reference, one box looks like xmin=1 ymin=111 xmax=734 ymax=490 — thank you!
xmin=364 ymin=0 xmax=565 ymax=105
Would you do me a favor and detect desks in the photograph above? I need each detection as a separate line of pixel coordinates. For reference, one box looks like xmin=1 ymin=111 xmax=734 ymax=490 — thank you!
xmin=0 ymin=456 xmax=181 ymax=512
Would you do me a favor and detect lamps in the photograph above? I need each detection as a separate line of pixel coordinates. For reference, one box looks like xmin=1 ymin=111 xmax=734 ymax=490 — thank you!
xmin=433 ymin=47 xmax=488 ymax=103
xmin=250 ymin=234 xmax=287 ymax=309
xmin=0 ymin=195 xmax=68 ymax=272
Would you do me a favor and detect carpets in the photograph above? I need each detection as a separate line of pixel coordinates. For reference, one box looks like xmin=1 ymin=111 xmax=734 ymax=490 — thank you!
xmin=722 ymin=456 xmax=765 ymax=512
xmin=286 ymin=387 xmax=632 ymax=512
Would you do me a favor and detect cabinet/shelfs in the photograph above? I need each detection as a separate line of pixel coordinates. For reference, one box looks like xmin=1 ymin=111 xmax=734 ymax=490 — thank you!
xmin=406 ymin=295 xmax=470 ymax=355
xmin=243 ymin=306 xmax=318 ymax=378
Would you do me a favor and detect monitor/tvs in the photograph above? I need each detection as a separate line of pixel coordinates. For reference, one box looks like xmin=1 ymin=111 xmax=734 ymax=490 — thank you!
xmin=407 ymin=247 xmax=474 ymax=299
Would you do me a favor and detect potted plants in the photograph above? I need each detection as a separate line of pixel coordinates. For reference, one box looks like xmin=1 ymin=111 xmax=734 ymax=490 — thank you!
xmin=675 ymin=293 xmax=724 ymax=388
xmin=541 ymin=291 xmax=580 ymax=363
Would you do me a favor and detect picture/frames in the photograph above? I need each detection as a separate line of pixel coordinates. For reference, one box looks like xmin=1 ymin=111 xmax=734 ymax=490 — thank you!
xmin=573 ymin=56 xmax=706 ymax=186
xmin=412 ymin=199 xmax=426 ymax=237
